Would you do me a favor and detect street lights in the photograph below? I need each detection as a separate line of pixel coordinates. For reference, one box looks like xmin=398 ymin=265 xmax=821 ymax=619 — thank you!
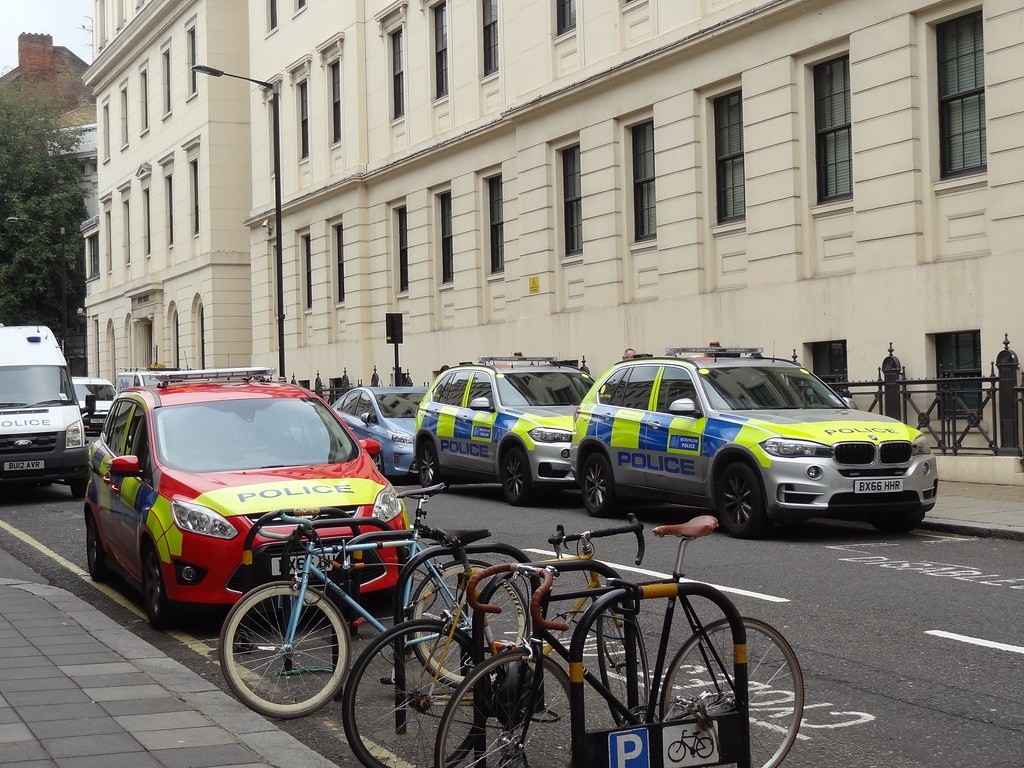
xmin=190 ymin=64 xmax=287 ymax=385
xmin=6 ymin=217 xmax=69 ymax=358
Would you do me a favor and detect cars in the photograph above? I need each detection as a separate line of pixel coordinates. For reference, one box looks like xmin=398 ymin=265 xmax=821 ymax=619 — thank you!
xmin=330 ymin=386 xmax=432 ymax=475
xmin=570 ymin=339 xmax=939 ymax=540
xmin=84 ymin=369 xmax=411 ymax=633
xmin=413 ymin=352 xmax=596 ymax=509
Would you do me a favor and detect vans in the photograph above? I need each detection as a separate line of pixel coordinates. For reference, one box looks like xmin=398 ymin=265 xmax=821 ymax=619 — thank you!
xmin=71 ymin=375 xmax=116 ymax=431
xmin=0 ymin=323 xmax=98 ymax=500
xmin=115 ymin=363 xmax=164 ymax=395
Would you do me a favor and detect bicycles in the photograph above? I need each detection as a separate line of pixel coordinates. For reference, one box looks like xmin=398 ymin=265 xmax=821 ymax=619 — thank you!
xmin=216 ymin=481 xmax=529 ymax=720
xmin=432 ymin=514 xmax=804 ymax=768
xmin=340 ymin=514 xmax=651 ymax=768
